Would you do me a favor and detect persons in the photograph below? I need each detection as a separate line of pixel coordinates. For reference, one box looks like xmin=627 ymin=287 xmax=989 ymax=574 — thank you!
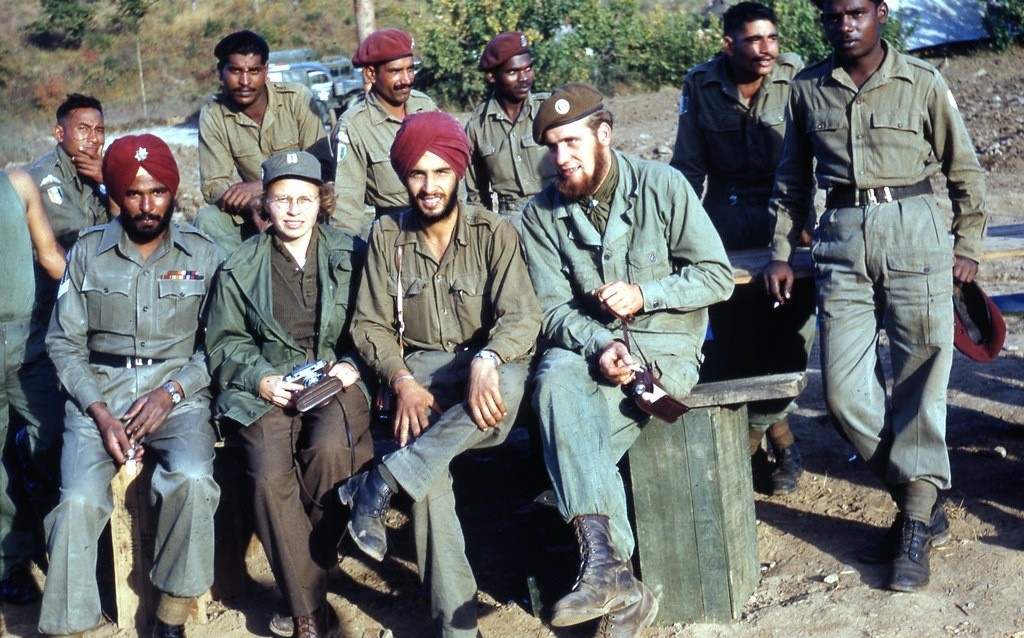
xmin=0 ymin=93 xmax=230 ymax=638
xmin=519 ymin=85 xmax=736 ymax=638
xmin=203 ymin=152 xmax=378 ymax=638
xmin=329 ymin=27 xmax=445 ymax=241
xmin=191 ymin=29 xmax=337 ymax=258
xmin=666 ymin=0 xmax=818 ymax=497
xmin=461 ymin=31 xmax=560 ymax=217
xmin=337 ymin=110 xmax=541 ymax=638
xmin=759 ymin=0 xmax=988 ymax=593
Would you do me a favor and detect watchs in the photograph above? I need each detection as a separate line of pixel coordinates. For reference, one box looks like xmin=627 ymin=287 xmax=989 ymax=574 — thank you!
xmin=160 ymin=382 xmax=182 ymax=405
xmin=472 ymin=350 xmax=499 ymax=370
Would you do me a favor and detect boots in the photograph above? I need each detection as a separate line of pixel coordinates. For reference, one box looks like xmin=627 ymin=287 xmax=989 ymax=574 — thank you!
xmin=550 ymin=514 xmax=642 ymax=627
xmin=591 ymin=560 xmax=660 ymax=638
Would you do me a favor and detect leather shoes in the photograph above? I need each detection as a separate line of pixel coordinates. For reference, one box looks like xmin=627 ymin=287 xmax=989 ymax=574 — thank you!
xmin=751 ymin=442 xmax=803 ymax=495
xmin=149 ymin=623 xmax=187 ymax=638
xmin=0 ymin=571 xmax=43 ymax=605
xmin=270 ymin=593 xmax=340 ymax=638
xmin=860 ymin=500 xmax=951 ymax=562
xmin=339 ymin=467 xmax=394 ymax=562
xmin=886 ymin=517 xmax=930 ymax=591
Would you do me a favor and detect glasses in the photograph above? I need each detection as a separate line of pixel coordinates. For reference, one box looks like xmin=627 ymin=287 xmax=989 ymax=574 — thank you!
xmin=268 ymin=196 xmax=317 ymax=212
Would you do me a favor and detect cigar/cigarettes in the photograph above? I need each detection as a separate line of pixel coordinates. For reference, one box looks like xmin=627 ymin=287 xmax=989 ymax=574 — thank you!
xmin=773 ymin=294 xmax=786 ymax=308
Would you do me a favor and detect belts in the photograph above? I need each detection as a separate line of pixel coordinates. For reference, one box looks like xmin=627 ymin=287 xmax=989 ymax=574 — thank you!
xmin=826 ymin=176 xmax=932 ymax=207
xmin=706 ymin=190 xmax=768 ymax=207
xmin=89 ymin=349 xmax=167 ymax=370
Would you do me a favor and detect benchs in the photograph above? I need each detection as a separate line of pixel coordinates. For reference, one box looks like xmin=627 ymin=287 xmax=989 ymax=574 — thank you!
xmin=109 ymin=369 xmax=806 ymax=626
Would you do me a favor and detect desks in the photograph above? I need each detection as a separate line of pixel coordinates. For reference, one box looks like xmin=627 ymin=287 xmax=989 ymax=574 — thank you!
xmin=723 ymin=242 xmax=817 ymax=287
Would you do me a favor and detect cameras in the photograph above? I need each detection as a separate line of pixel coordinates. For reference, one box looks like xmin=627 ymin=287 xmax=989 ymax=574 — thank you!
xmin=286 ymin=358 xmax=327 ymax=387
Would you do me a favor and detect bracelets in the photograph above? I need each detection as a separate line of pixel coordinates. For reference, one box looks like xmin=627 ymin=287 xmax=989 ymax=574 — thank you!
xmin=391 ymin=375 xmax=415 ymax=386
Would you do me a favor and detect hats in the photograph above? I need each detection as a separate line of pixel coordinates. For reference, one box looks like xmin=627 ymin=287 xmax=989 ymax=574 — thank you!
xmin=390 ymin=112 xmax=471 ymax=182
xmin=261 ymin=152 xmax=325 ymax=186
xmin=351 ymin=29 xmax=413 ymax=68
xmin=477 ymin=33 xmax=527 ymax=71
xmin=532 ymin=85 xmax=604 ymax=147
xmin=954 ymin=275 xmax=1006 ymax=361
xmin=102 ymin=134 xmax=180 ymax=206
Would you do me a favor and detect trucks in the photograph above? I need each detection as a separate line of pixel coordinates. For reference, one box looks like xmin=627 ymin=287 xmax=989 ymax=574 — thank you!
xmin=266 ymin=50 xmax=365 ymax=111
